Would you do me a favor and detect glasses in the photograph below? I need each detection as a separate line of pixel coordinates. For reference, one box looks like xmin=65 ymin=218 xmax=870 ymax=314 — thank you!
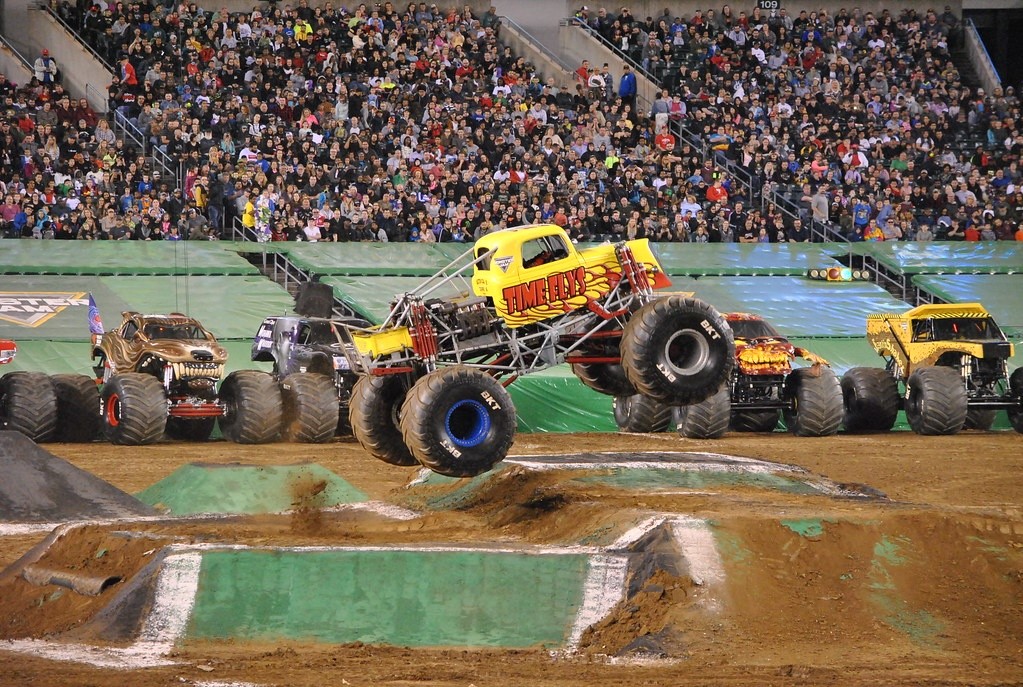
xmin=355 ymin=204 xmax=360 ymax=208
xmin=313 ymin=211 xmax=319 ymax=214
xmin=888 ymin=221 xmax=893 ymax=224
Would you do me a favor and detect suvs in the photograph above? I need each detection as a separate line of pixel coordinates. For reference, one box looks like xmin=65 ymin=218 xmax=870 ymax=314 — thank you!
xmin=45 ymin=308 xmax=283 ymax=444
xmin=610 ymin=309 xmax=844 ymax=442
xmin=842 ymin=303 xmax=1023 ymax=435
xmin=347 ymin=220 xmax=737 ymax=486
xmin=247 ymin=310 xmax=384 ymax=449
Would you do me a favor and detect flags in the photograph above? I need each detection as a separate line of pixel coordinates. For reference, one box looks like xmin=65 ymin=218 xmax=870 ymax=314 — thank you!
xmin=88 ymin=293 xmax=105 ymax=335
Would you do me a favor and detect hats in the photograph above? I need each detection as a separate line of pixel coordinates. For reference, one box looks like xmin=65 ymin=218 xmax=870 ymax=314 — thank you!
xmin=68 ymin=134 xmax=77 ymax=139
xmin=852 ymin=144 xmax=859 ymax=149
xmin=410 ymin=192 xmax=417 ymax=196
xmin=588 ymin=68 xmax=593 ymax=73
xmin=320 ymin=44 xmax=326 ymax=48
xmin=661 ymin=124 xmax=667 ymax=129
xmin=318 ymin=75 xmax=326 ymax=82
xmin=174 ymin=188 xmax=181 ymax=192
xmin=933 ymin=188 xmax=939 ymax=193
xmin=354 ymin=200 xmax=360 ymax=204
xmin=400 ymin=166 xmax=408 ymax=172
xmin=170 ymin=34 xmax=177 ymax=40
xmin=623 ymin=65 xmax=630 ymax=70
xmin=921 ymin=170 xmax=928 ymax=174
xmin=42 ymin=48 xmax=49 ymax=55
xmin=876 ymin=71 xmax=884 ymax=77
xmin=499 ymin=163 xmax=507 ymax=171
xmin=615 ymin=96 xmax=621 ymax=100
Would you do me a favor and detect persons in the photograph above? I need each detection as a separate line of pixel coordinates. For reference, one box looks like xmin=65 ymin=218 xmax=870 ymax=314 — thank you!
xmin=798 ymin=84 xmax=1023 ymax=241
xmin=522 ymin=58 xmax=812 ymax=243
xmin=145 ymin=109 xmax=522 ymax=242
xmin=47 ymin=0 xmax=523 ymax=118
xmin=573 ymin=5 xmax=964 ymax=94
xmin=0 ymin=48 xmax=221 ymax=240
